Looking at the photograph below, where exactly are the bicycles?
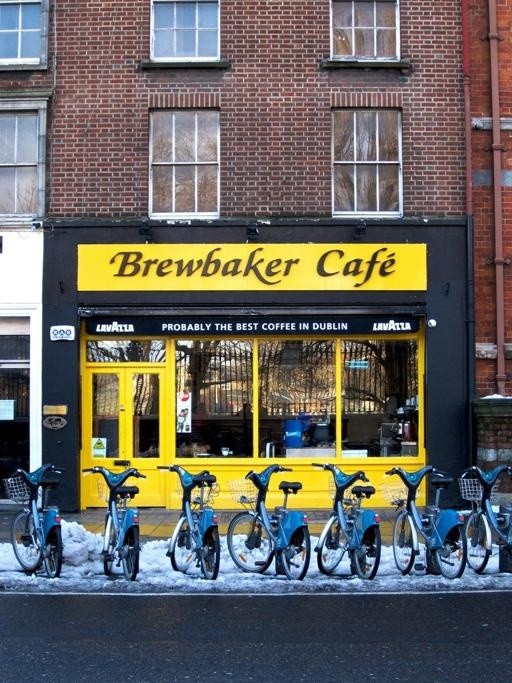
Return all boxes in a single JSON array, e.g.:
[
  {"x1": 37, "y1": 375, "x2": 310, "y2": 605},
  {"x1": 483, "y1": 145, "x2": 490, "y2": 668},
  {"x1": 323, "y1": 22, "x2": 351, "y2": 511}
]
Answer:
[
  {"x1": 387, "y1": 463, "x2": 468, "y2": 579},
  {"x1": 3, "y1": 462, "x2": 65, "y2": 579},
  {"x1": 158, "y1": 465, "x2": 221, "y2": 580},
  {"x1": 313, "y1": 461, "x2": 381, "y2": 580},
  {"x1": 83, "y1": 465, "x2": 146, "y2": 578},
  {"x1": 227, "y1": 464, "x2": 310, "y2": 581},
  {"x1": 459, "y1": 465, "x2": 512, "y2": 573}
]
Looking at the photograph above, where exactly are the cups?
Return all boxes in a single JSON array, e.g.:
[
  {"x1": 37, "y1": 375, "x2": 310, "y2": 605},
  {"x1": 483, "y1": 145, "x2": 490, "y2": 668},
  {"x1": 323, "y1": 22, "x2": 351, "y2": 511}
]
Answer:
[{"x1": 221, "y1": 447, "x2": 229, "y2": 457}]
